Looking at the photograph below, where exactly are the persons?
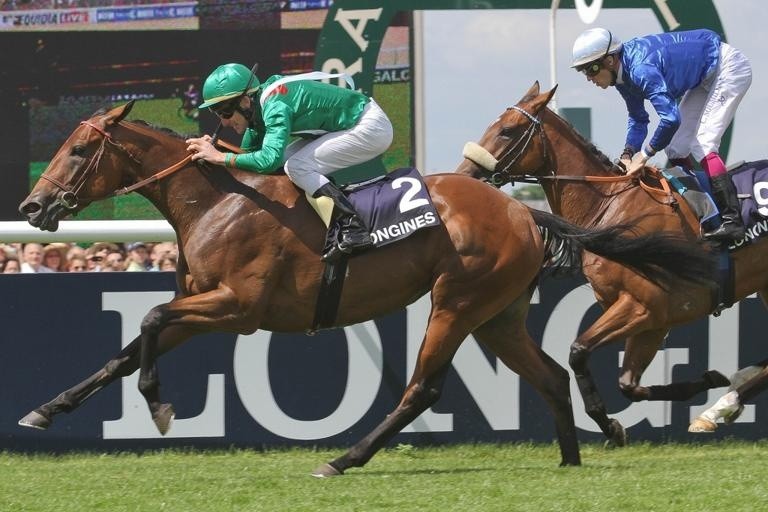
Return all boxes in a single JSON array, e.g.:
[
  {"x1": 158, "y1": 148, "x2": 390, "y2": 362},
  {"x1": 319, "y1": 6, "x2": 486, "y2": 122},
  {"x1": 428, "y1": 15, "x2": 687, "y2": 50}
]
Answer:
[
  {"x1": 183, "y1": 61, "x2": 395, "y2": 263},
  {"x1": 0, "y1": 242, "x2": 179, "y2": 274},
  {"x1": 568, "y1": 27, "x2": 752, "y2": 241}
]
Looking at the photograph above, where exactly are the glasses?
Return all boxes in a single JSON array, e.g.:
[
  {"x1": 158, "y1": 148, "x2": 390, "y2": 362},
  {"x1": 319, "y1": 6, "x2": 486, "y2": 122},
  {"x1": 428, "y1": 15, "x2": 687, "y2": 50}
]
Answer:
[
  {"x1": 213, "y1": 97, "x2": 243, "y2": 118},
  {"x1": 581, "y1": 62, "x2": 609, "y2": 78}
]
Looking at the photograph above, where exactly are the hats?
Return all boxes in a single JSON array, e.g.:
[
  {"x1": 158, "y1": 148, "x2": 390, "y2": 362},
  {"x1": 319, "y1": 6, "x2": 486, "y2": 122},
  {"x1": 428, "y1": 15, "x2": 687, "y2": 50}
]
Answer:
[{"x1": 128, "y1": 241, "x2": 146, "y2": 251}]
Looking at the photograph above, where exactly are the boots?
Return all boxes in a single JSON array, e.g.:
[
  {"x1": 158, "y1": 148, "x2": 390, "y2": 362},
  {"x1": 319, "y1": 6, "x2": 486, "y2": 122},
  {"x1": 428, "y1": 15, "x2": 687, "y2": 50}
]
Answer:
[
  {"x1": 703, "y1": 171, "x2": 746, "y2": 241},
  {"x1": 312, "y1": 182, "x2": 374, "y2": 264}
]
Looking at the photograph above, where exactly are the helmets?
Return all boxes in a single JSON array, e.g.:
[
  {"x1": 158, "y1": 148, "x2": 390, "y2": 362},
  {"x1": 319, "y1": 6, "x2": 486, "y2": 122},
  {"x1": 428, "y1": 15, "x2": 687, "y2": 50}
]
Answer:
[
  {"x1": 199, "y1": 63, "x2": 260, "y2": 109},
  {"x1": 570, "y1": 28, "x2": 622, "y2": 68}
]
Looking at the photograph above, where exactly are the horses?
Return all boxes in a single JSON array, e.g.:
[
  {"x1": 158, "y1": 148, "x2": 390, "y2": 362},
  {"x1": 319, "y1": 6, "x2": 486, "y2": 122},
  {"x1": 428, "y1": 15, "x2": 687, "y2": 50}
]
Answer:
[
  {"x1": 18, "y1": 98, "x2": 739, "y2": 479},
  {"x1": 452, "y1": 80, "x2": 768, "y2": 449}
]
